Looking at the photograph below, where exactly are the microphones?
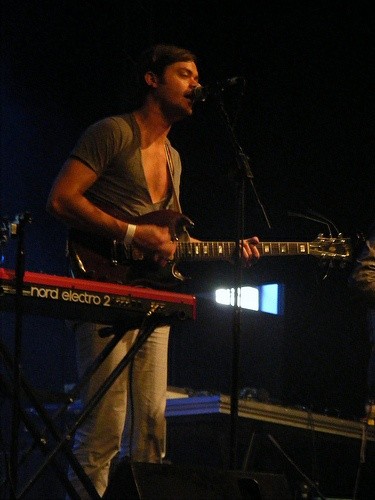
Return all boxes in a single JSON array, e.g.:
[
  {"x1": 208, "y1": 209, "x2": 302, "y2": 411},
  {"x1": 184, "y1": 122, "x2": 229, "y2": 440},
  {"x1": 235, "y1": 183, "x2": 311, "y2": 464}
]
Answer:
[
  {"x1": 8, "y1": 210, "x2": 32, "y2": 228},
  {"x1": 192, "y1": 77, "x2": 239, "y2": 101}
]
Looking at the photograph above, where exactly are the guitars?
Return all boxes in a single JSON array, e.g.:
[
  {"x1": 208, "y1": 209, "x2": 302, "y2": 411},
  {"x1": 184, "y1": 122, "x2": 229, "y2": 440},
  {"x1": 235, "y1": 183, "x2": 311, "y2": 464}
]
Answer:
[{"x1": 68, "y1": 206, "x2": 355, "y2": 282}]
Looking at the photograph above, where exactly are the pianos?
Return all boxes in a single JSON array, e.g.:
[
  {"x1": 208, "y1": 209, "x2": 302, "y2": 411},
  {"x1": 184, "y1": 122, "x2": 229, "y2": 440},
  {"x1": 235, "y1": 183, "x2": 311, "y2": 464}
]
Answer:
[{"x1": 0, "y1": 266, "x2": 209, "y2": 325}]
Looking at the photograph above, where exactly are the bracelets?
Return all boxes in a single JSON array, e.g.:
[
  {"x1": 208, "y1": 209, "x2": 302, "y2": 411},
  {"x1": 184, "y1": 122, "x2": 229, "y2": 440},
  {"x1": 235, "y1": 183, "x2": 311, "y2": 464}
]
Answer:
[{"x1": 124, "y1": 224, "x2": 136, "y2": 244}]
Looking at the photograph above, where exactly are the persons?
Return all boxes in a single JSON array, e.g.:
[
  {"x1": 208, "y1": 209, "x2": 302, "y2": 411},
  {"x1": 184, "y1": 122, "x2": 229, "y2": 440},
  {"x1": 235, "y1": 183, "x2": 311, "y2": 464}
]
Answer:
[{"x1": 46, "y1": 45, "x2": 261, "y2": 500}]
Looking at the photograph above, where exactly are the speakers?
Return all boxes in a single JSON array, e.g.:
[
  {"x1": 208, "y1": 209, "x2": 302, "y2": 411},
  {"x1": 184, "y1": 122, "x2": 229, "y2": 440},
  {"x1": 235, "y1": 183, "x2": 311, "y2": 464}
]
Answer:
[{"x1": 101, "y1": 460, "x2": 292, "y2": 500}]
